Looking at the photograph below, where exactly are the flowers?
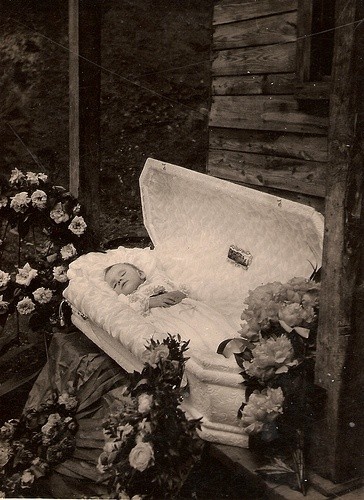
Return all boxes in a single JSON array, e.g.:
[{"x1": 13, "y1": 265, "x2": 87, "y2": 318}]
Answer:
[
  {"x1": 229, "y1": 277, "x2": 320, "y2": 446},
  {"x1": 93, "y1": 332, "x2": 204, "y2": 498},
  {"x1": 0, "y1": 168, "x2": 90, "y2": 342},
  {"x1": 0, "y1": 389, "x2": 81, "y2": 500}
]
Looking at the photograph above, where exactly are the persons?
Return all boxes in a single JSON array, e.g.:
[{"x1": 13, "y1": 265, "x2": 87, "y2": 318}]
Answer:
[{"x1": 102, "y1": 262, "x2": 247, "y2": 360}]
